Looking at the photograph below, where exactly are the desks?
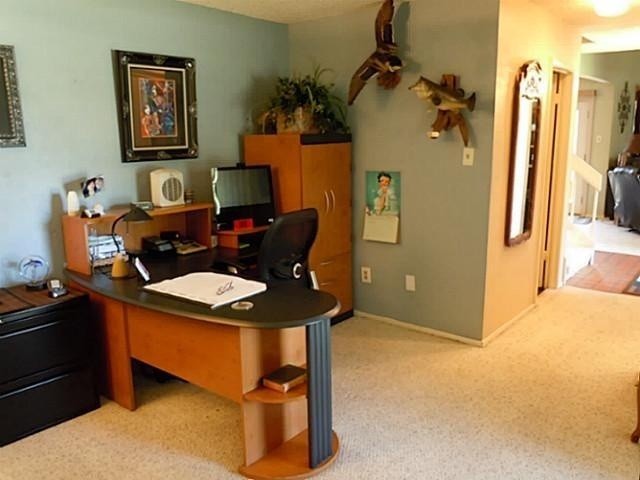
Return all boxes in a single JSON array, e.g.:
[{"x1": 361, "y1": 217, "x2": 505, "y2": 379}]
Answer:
[{"x1": 53, "y1": 191, "x2": 342, "y2": 479}]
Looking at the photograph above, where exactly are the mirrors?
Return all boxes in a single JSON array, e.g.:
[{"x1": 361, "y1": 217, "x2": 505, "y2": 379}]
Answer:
[{"x1": 504, "y1": 58, "x2": 547, "y2": 246}]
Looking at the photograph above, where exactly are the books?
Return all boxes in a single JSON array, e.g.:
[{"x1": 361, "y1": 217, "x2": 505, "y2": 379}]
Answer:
[
  {"x1": 141, "y1": 271, "x2": 267, "y2": 311},
  {"x1": 261, "y1": 364, "x2": 306, "y2": 393}
]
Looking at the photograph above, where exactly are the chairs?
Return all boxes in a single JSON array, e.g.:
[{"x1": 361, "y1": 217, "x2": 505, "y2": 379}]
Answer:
[{"x1": 227, "y1": 207, "x2": 321, "y2": 293}]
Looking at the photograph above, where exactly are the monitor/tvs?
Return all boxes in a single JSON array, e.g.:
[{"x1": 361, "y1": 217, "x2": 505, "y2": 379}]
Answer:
[{"x1": 211, "y1": 164, "x2": 275, "y2": 225}]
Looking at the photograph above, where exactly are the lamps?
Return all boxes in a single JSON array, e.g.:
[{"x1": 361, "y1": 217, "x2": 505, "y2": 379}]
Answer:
[{"x1": 109, "y1": 202, "x2": 157, "y2": 278}]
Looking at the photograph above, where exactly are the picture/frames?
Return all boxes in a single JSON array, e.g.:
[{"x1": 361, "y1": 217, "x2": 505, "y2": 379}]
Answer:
[
  {"x1": 0, "y1": 41, "x2": 29, "y2": 152},
  {"x1": 112, "y1": 46, "x2": 202, "y2": 165}
]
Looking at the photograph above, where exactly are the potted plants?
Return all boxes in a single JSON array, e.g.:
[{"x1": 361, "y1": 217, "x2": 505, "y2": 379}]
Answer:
[{"x1": 265, "y1": 61, "x2": 350, "y2": 138}]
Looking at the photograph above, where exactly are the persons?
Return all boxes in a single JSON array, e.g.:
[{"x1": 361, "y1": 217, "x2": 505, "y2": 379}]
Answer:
[{"x1": 372, "y1": 171, "x2": 395, "y2": 216}]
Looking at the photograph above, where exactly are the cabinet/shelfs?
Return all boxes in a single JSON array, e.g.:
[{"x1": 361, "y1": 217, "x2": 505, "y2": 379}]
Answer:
[
  {"x1": 1, "y1": 280, "x2": 103, "y2": 449},
  {"x1": 212, "y1": 223, "x2": 272, "y2": 260},
  {"x1": 307, "y1": 256, "x2": 356, "y2": 327},
  {"x1": 239, "y1": 130, "x2": 355, "y2": 261}
]
